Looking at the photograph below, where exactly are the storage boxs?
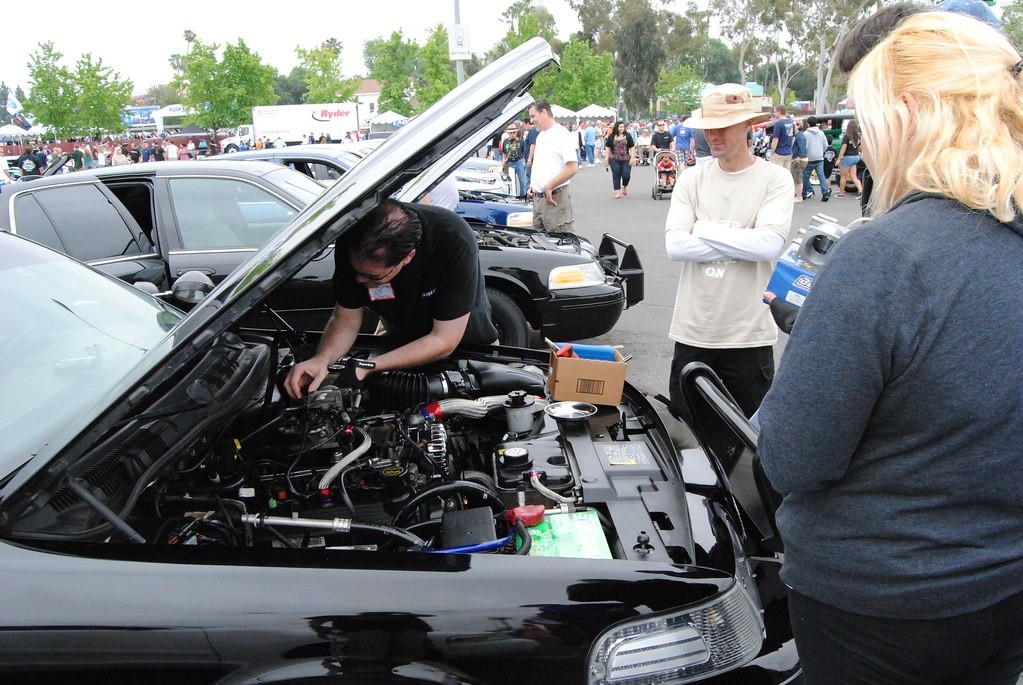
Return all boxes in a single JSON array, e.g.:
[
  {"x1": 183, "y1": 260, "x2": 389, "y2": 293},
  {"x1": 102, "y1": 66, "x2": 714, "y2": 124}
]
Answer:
[
  {"x1": 548, "y1": 342, "x2": 627, "y2": 406},
  {"x1": 761, "y1": 237, "x2": 817, "y2": 310}
]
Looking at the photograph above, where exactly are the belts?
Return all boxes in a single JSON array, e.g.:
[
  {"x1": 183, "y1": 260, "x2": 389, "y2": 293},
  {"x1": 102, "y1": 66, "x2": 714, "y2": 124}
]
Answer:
[{"x1": 534, "y1": 185, "x2": 567, "y2": 198}]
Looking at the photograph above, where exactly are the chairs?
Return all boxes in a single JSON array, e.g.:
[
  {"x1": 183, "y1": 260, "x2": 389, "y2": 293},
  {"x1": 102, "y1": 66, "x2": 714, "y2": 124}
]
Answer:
[{"x1": 182, "y1": 205, "x2": 243, "y2": 249}]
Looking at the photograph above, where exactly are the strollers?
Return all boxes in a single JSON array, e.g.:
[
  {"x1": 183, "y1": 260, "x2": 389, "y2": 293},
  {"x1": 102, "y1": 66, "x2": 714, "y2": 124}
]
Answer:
[{"x1": 651, "y1": 150, "x2": 679, "y2": 201}]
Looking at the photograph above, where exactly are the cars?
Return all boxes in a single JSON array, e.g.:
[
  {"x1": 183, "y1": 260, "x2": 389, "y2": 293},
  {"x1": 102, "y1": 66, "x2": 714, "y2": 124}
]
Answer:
[{"x1": 0, "y1": 34, "x2": 804, "y2": 685}]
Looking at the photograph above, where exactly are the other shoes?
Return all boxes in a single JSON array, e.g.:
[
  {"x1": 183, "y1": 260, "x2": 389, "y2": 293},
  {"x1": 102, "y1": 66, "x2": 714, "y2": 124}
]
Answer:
[
  {"x1": 835, "y1": 194, "x2": 844, "y2": 199},
  {"x1": 622, "y1": 188, "x2": 627, "y2": 195},
  {"x1": 577, "y1": 158, "x2": 603, "y2": 170},
  {"x1": 614, "y1": 194, "x2": 620, "y2": 199},
  {"x1": 854, "y1": 191, "x2": 862, "y2": 200},
  {"x1": 793, "y1": 187, "x2": 834, "y2": 204}
]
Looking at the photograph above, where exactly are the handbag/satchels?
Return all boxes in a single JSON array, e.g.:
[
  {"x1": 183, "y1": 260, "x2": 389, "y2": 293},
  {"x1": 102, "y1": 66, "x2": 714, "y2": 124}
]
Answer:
[{"x1": 501, "y1": 160, "x2": 508, "y2": 174}]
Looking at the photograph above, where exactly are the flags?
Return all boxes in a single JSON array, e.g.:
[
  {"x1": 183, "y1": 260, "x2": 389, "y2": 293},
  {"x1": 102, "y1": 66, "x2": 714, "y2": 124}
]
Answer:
[{"x1": 6, "y1": 93, "x2": 32, "y2": 131}]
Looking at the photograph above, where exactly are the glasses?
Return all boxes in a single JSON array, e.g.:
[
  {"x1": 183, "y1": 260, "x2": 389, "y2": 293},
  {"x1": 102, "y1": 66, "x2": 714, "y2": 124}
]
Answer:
[
  {"x1": 658, "y1": 124, "x2": 666, "y2": 126},
  {"x1": 345, "y1": 267, "x2": 396, "y2": 281}
]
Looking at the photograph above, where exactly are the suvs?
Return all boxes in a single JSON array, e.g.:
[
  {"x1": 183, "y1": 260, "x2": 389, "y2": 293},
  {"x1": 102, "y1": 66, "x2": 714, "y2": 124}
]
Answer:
[{"x1": 804, "y1": 114, "x2": 863, "y2": 193}]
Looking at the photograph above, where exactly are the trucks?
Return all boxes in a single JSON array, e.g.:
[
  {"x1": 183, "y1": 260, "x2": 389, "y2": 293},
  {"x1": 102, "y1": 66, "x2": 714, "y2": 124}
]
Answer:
[{"x1": 219, "y1": 101, "x2": 358, "y2": 153}]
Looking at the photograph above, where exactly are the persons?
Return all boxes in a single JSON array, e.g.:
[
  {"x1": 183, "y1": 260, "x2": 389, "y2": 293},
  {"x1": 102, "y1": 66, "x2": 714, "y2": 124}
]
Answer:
[
  {"x1": 527, "y1": 100, "x2": 579, "y2": 234},
  {"x1": 303, "y1": 129, "x2": 370, "y2": 145},
  {"x1": 417, "y1": 171, "x2": 460, "y2": 210},
  {"x1": 756, "y1": 10, "x2": 1023, "y2": 685},
  {"x1": 284, "y1": 198, "x2": 499, "y2": 402},
  {"x1": 770, "y1": 104, "x2": 795, "y2": 171},
  {"x1": 523, "y1": 565, "x2": 733, "y2": 654},
  {"x1": 665, "y1": 83, "x2": 794, "y2": 472},
  {"x1": 486, "y1": 115, "x2": 865, "y2": 203},
  {"x1": 0, "y1": 126, "x2": 286, "y2": 186},
  {"x1": 838, "y1": 0, "x2": 1002, "y2": 83}
]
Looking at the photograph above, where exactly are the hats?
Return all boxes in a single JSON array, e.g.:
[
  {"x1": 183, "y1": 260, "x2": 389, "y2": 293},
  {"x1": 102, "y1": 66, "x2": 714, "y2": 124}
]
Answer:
[
  {"x1": 681, "y1": 83, "x2": 772, "y2": 130},
  {"x1": 506, "y1": 123, "x2": 520, "y2": 132}
]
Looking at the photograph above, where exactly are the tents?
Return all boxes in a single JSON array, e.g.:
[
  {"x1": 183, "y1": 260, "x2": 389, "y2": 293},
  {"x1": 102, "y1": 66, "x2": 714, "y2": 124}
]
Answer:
[
  {"x1": 0, "y1": 112, "x2": 106, "y2": 144},
  {"x1": 549, "y1": 104, "x2": 617, "y2": 126},
  {"x1": 171, "y1": 123, "x2": 214, "y2": 137},
  {"x1": 369, "y1": 111, "x2": 408, "y2": 133}
]
